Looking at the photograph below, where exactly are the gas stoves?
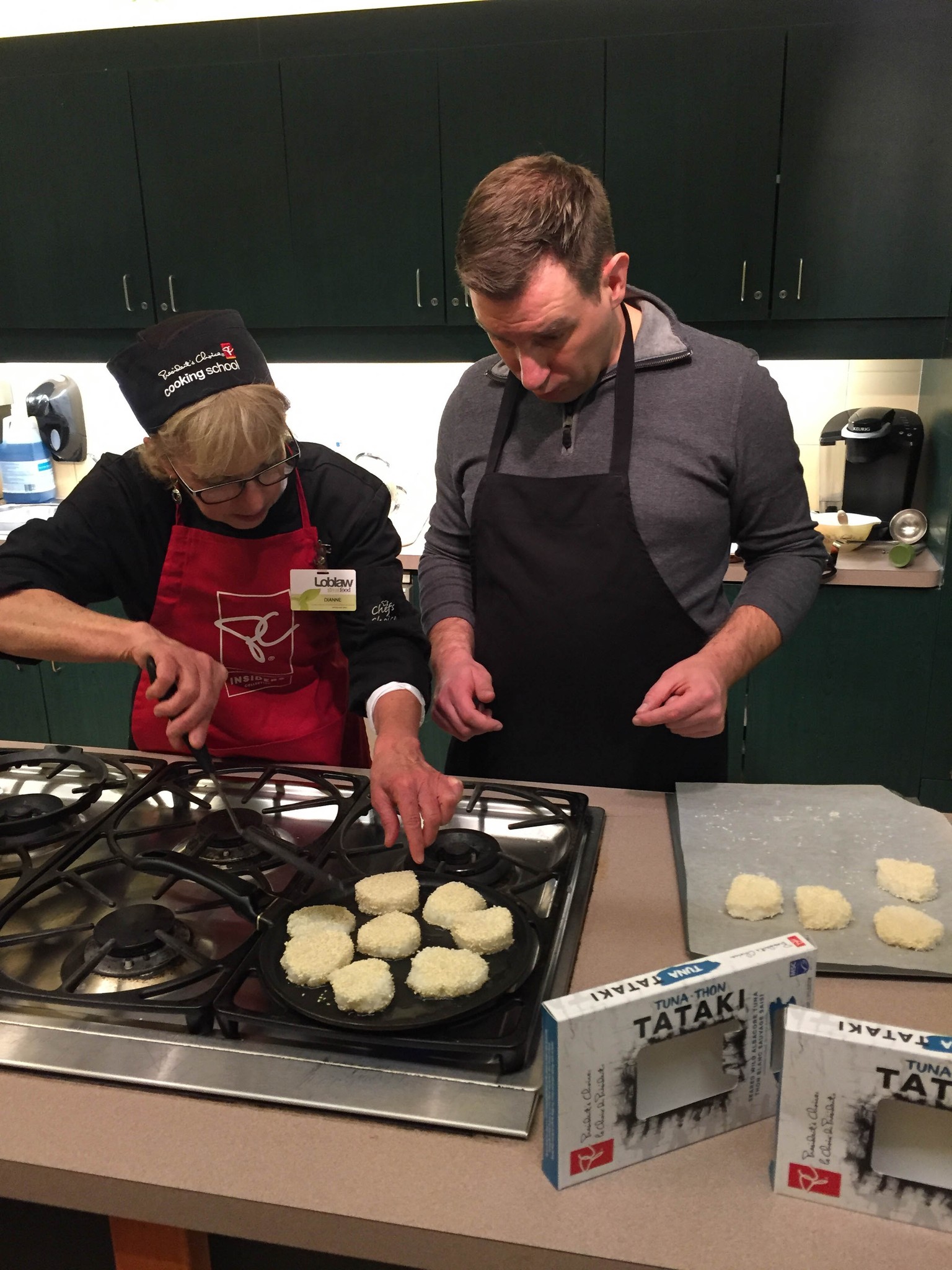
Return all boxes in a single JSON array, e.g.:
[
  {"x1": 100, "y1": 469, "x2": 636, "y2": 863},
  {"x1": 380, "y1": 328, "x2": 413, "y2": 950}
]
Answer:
[{"x1": 0, "y1": 731, "x2": 618, "y2": 1152}]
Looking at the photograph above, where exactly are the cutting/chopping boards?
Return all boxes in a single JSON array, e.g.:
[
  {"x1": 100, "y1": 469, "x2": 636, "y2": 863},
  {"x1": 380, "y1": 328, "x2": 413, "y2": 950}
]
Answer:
[{"x1": 667, "y1": 786, "x2": 952, "y2": 984}]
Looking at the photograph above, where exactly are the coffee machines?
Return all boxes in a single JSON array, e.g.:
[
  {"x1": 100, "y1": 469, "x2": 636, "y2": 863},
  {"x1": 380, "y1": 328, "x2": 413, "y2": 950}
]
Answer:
[{"x1": 819, "y1": 405, "x2": 925, "y2": 540}]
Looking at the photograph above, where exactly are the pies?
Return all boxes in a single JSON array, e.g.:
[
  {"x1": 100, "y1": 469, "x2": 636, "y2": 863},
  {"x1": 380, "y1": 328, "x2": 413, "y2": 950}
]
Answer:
[
  {"x1": 280, "y1": 867, "x2": 514, "y2": 1015},
  {"x1": 728, "y1": 854, "x2": 947, "y2": 953}
]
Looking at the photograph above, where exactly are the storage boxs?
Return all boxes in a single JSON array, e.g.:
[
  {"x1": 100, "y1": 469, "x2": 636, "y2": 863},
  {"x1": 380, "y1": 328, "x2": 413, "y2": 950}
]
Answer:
[
  {"x1": 773, "y1": 1001, "x2": 952, "y2": 1235},
  {"x1": 540, "y1": 932, "x2": 817, "y2": 1193}
]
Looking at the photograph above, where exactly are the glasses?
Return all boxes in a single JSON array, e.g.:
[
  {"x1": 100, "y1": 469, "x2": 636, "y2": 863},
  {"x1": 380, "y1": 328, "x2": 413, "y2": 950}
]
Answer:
[{"x1": 163, "y1": 422, "x2": 303, "y2": 504}]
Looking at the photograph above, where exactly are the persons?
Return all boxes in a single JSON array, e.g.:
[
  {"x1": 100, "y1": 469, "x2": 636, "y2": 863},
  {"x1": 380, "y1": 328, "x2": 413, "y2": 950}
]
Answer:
[
  {"x1": 0, "y1": 307, "x2": 466, "y2": 864},
  {"x1": 415, "y1": 152, "x2": 829, "y2": 791}
]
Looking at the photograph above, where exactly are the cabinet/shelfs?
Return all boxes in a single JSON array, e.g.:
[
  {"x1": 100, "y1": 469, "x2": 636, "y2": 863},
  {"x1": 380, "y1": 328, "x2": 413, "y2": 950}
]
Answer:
[
  {"x1": 1, "y1": 595, "x2": 139, "y2": 749},
  {"x1": 603, "y1": 16, "x2": 951, "y2": 322},
  {"x1": 0, "y1": 58, "x2": 298, "y2": 331},
  {"x1": 277, "y1": 36, "x2": 604, "y2": 329},
  {"x1": 717, "y1": 580, "x2": 942, "y2": 809}
]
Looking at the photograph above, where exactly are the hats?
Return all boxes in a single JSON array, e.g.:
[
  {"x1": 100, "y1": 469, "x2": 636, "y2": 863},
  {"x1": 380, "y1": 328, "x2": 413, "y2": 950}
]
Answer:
[{"x1": 104, "y1": 310, "x2": 275, "y2": 436}]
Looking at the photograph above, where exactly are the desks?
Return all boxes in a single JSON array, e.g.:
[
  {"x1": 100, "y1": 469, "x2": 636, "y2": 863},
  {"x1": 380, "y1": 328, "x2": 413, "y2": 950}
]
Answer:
[{"x1": 1, "y1": 740, "x2": 951, "y2": 1270}]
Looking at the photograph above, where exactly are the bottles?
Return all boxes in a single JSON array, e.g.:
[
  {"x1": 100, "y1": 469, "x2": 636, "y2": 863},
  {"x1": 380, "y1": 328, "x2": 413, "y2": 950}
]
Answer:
[{"x1": 0, "y1": 403, "x2": 58, "y2": 505}]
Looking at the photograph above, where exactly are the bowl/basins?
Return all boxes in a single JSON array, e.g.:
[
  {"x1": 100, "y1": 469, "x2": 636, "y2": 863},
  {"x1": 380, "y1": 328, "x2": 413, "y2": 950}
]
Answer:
[{"x1": 810, "y1": 508, "x2": 884, "y2": 554}]
